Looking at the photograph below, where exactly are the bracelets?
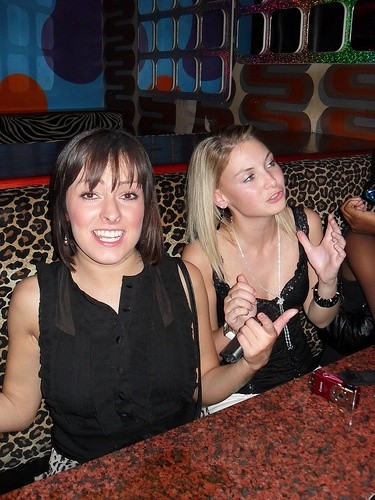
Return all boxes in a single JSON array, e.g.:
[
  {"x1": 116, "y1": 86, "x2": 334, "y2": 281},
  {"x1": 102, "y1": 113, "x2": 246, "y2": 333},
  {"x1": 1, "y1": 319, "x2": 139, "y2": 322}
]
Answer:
[{"x1": 313, "y1": 282, "x2": 340, "y2": 307}]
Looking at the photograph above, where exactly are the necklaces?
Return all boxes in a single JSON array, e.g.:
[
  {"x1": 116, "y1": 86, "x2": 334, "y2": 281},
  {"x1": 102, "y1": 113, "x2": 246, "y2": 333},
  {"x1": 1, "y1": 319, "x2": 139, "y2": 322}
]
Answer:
[{"x1": 230, "y1": 216, "x2": 294, "y2": 350}]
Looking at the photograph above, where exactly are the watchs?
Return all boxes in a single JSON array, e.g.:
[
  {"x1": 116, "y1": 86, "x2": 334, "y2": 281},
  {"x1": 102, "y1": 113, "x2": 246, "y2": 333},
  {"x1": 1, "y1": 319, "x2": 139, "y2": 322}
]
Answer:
[{"x1": 224, "y1": 322, "x2": 236, "y2": 340}]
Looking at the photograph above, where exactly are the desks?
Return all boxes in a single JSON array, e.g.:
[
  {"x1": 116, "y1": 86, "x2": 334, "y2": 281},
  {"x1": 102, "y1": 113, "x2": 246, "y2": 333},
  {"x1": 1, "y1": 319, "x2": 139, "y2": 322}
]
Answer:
[{"x1": 0, "y1": 343, "x2": 375, "y2": 500}]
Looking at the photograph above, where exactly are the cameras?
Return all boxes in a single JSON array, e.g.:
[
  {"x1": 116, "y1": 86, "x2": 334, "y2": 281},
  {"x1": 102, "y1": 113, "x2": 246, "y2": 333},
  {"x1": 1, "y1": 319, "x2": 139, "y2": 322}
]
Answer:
[{"x1": 308, "y1": 369, "x2": 361, "y2": 411}]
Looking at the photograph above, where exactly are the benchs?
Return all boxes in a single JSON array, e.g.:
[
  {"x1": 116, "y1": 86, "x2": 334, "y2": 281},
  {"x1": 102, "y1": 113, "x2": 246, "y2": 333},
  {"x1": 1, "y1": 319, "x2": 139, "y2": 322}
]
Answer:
[{"x1": 0, "y1": 154, "x2": 375, "y2": 493}]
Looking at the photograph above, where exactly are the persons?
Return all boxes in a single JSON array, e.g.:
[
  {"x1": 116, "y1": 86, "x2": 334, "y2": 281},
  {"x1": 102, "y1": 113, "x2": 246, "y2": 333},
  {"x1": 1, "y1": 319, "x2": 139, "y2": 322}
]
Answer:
[
  {"x1": 181, "y1": 125, "x2": 347, "y2": 415},
  {"x1": 0, "y1": 128, "x2": 299, "y2": 477},
  {"x1": 342, "y1": 197, "x2": 375, "y2": 316}
]
